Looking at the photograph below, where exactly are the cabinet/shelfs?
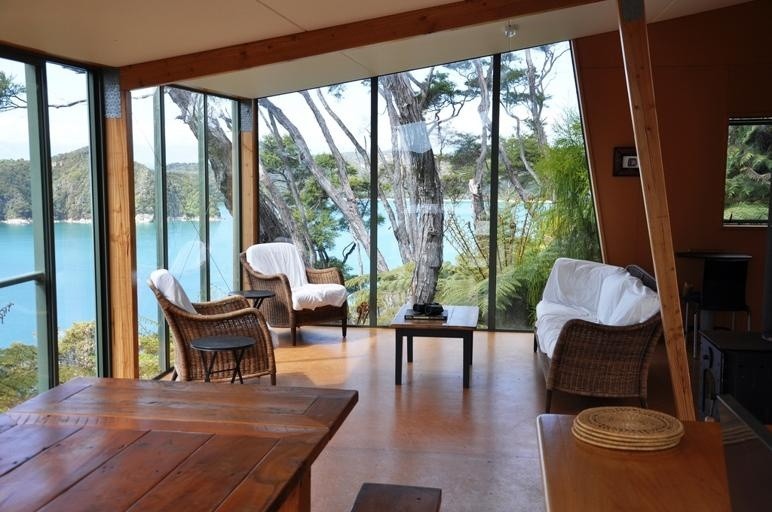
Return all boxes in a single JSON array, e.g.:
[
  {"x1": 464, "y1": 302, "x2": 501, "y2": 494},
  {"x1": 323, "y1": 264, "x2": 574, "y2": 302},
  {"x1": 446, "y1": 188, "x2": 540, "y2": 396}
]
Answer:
[{"x1": 697, "y1": 329, "x2": 772, "y2": 423}]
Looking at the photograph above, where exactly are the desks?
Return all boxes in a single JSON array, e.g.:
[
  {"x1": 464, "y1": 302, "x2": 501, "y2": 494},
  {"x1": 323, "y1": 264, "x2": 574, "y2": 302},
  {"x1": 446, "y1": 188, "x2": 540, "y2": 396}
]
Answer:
[
  {"x1": 537, "y1": 414, "x2": 732, "y2": 512},
  {"x1": 2, "y1": 371, "x2": 357, "y2": 512}
]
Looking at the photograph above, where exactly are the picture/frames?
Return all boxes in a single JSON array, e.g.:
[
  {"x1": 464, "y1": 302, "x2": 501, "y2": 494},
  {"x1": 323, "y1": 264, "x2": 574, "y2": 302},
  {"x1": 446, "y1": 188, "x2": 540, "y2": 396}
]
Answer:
[{"x1": 613, "y1": 147, "x2": 640, "y2": 178}]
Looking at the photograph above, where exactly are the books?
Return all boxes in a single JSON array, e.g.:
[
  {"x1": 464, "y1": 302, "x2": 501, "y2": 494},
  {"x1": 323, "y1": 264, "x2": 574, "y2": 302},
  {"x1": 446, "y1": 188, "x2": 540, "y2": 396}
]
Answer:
[{"x1": 404, "y1": 309, "x2": 448, "y2": 320}]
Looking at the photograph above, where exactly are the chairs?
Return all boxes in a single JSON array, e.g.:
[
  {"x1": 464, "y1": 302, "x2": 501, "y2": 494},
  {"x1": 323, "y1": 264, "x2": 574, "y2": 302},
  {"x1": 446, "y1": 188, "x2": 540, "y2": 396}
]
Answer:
[
  {"x1": 147, "y1": 242, "x2": 348, "y2": 387},
  {"x1": 675, "y1": 251, "x2": 753, "y2": 360}
]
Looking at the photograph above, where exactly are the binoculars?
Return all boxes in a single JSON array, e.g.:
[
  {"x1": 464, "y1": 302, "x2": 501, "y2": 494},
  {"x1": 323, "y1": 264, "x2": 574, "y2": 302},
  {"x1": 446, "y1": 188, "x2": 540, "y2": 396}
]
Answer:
[{"x1": 413, "y1": 303, "x2": 443, "y2": 316}]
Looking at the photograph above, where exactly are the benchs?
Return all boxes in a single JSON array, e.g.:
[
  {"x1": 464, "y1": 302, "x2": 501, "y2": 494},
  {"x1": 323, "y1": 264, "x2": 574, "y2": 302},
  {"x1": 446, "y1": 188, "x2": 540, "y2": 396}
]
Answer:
[{"x1": 533, "y1": 266, "x2": 664, "y2": 414}]
